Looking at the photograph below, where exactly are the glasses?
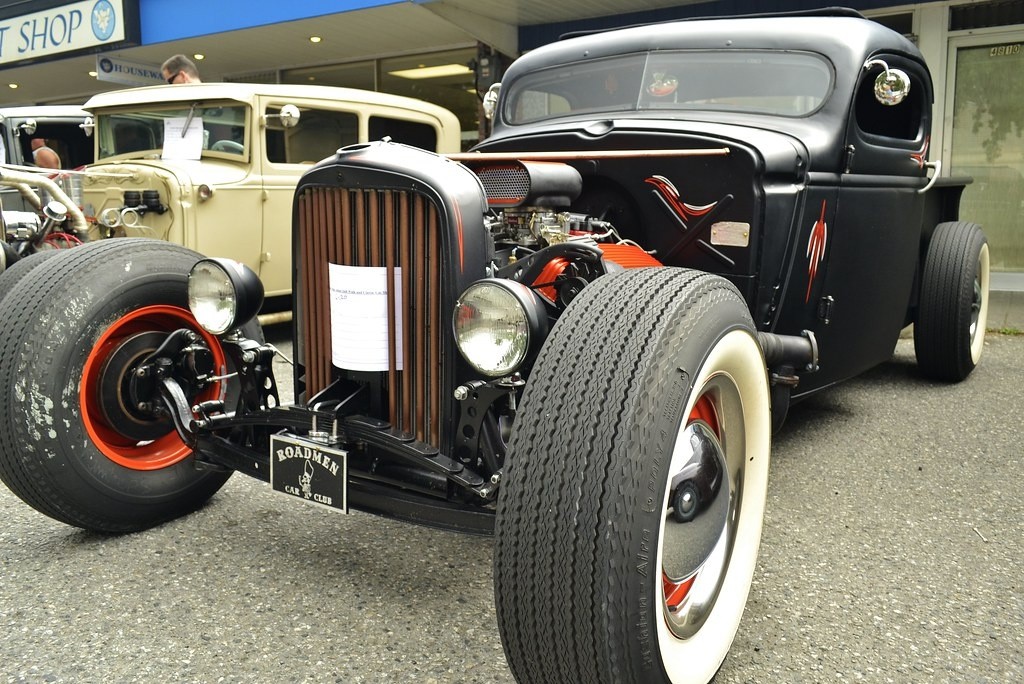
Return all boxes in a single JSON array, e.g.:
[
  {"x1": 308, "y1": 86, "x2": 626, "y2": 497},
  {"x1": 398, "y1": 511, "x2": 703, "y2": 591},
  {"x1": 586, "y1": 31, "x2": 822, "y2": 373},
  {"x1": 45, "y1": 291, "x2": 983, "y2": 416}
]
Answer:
[{"x1": 166, "y1": 72, "x2": 180, "y2": 84}]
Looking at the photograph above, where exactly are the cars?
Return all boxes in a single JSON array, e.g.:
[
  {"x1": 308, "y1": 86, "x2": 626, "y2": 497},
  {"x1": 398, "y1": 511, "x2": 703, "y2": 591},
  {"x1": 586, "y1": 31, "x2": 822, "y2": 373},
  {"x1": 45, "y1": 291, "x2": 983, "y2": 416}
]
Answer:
[
  {"x1": 0, "y1": 80, "x2": 463, "y2": 352},
  {"x1": 0, "y1": 3, "x2": 994, "y2": 683},
  {"x1": 0, "y1": 104, "x2": 167, "y2": 218}
]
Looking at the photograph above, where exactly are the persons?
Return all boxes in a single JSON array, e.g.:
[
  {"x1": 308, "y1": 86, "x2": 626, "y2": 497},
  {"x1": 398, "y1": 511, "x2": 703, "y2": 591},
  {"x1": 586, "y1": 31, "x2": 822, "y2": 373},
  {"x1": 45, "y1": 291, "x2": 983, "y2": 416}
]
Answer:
[
  {"x1": 161, "y1": 54, "x2": 201, "y2": 85},
  {"x1": 30, "y1": 139, "x2": 61, "y2": 170}
]
